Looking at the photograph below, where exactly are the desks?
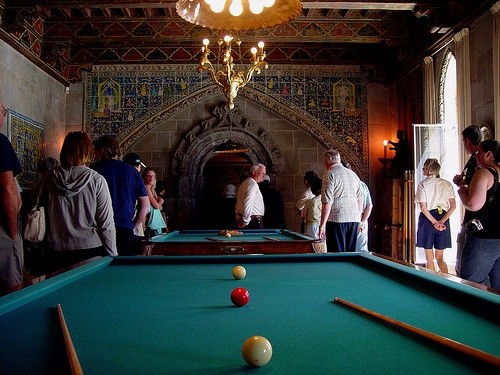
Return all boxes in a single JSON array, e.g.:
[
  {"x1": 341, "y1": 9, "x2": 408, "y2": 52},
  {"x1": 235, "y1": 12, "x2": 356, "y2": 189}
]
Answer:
[
  {"x1": 146, "y1": 231, "x2": 318, "y2": 255},
  {"x1": 0, "y1": 254, "x2": 500, "y2": 375}
]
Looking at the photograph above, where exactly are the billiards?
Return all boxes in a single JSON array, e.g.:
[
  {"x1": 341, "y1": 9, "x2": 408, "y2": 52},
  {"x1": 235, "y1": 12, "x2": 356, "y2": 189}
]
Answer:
[
  {"x1": 243, "y1": 336, "x2": 272, "y2": 366},
  {"x1": 233, "y1": 266, "x2": 246, "y2": 279},
  {"x1": 226, "y1": 233, "x2": 231, "y2": 238},
  {"x1": 231, "y1": 287, "x2": 250, "y2": 306},
  {"x1": 221, "y1": 230, "x2": 240, "y2": 233}
]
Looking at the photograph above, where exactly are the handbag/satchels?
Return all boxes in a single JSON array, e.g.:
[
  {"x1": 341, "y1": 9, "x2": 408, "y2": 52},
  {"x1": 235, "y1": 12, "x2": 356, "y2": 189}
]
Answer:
[{"x1": 24, "y1": 205, "x2": 45, "y2": 242}]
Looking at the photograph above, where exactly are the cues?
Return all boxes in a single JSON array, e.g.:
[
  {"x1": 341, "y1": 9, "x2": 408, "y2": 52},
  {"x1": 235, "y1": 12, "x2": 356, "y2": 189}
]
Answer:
[
  {"x1": 57, "y1": 303, "x2": 84, "y2": 375},
  {"x1": 206, "y1": 237, "x2": 224, "y2": 241},
  {"x1": 263, "y1": 236, "x2": 281, "y2": 241},
  {"x1": 402, "y1": 170, "x2": 414, "y2": 263},
  {"x1": 334, "y1": 296, "x2": 500, "y2": 373}
]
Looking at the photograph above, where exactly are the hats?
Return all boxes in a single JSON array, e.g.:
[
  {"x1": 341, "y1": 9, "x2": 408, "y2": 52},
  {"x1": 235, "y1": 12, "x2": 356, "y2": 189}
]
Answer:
[{"x1": 123, "y1": 153, "x2": 146, "y2": 168}]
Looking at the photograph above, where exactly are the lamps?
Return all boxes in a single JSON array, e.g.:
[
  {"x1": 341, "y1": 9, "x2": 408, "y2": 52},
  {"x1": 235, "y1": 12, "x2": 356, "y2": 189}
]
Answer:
[
  {"x1": 176, "y1": 0, "x2": 303, "y2": 31},
  {"x1": 196, "y1": 30, "x2": 268, "y2": 110}
]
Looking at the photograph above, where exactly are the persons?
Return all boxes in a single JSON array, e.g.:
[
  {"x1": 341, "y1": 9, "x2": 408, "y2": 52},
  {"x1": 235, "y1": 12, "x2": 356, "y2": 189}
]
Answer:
[
  {"x1": 457, "y1": 140, "x2": 500, "y2": 289},
  {"x1": 123, "y1": 153, "x2": 146, "y2": 242},
  {"x1": 142, "y1": 167, "x2": 172, "y2": 255},
  {"x1": 235, "y1": 163, "x2": 266, "y2": 229},
  {"x1": 0, "y1": 105, "x2": 24, "y2": 285},
  {"x1": 304, "y1": 178, "x2": 323, "y2": 243},
  {"x1": 42, "y1": 132, "x2": 119, "y2": 268},
  {"x1": 295, "y1": 170, "x2": 318, "y2": 233},
  {"x1": 414, "y1": 159, "x2": 457, "y2": 273},
  {"x1": 453, "y1": 125, "x2": 480, "y2": 277},
  {"x1": 221, "y1": 184, "x2": 237, "y2": 229},
  {"x1": 341, "y1": 162, "x2": 373, "y2": 252},
  {"x1": 260, "y1": 175, "x2": 287, "y2": 229},
  {"x1": 89, "y1": 135, "x2": 150, "y2": 256},
  {"x1": 318, "y1": 150, "x2": 365, "y2": 253}
]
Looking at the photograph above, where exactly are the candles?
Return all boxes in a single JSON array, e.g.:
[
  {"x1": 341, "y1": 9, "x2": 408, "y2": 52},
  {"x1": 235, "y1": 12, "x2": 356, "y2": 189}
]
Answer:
[{"x1": 383, "y1": 140, "x2": 388, "y2": 158}]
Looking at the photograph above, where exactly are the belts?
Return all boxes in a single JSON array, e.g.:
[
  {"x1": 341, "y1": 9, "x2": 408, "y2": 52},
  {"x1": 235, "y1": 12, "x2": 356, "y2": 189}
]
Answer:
[{"x1": 250, "y1": 215, "x2": 263, "y2": 219}]
